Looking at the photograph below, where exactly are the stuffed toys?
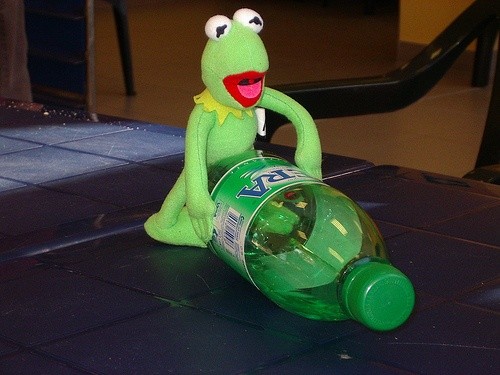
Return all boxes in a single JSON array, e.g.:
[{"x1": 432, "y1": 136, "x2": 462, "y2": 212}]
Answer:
[{"x1": 144, "y1": 9, "x2": 323, "y2": 247}]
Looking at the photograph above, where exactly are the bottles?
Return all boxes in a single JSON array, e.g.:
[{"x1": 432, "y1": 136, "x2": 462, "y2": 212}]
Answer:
[{"x1": 202, "y1": 148, "x2": 416, "y2": 332}]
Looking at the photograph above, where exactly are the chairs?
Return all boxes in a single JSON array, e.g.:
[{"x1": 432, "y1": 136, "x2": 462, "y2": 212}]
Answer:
[{"x1": 253, "y1": 1, "x2": 499, "y2": 185}]
